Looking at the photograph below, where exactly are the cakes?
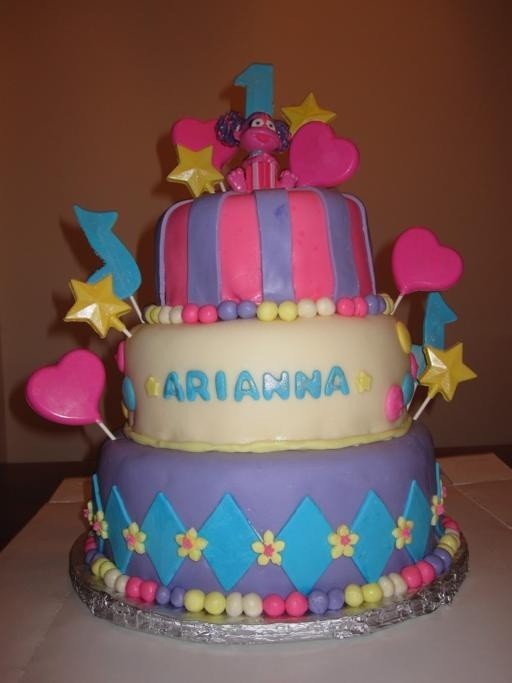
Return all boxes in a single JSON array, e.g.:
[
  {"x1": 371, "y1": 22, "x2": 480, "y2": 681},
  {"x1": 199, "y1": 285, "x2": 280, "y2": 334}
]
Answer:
[{"x1": 24, "y1": 60, "x2": 479, "y2": 620}]
